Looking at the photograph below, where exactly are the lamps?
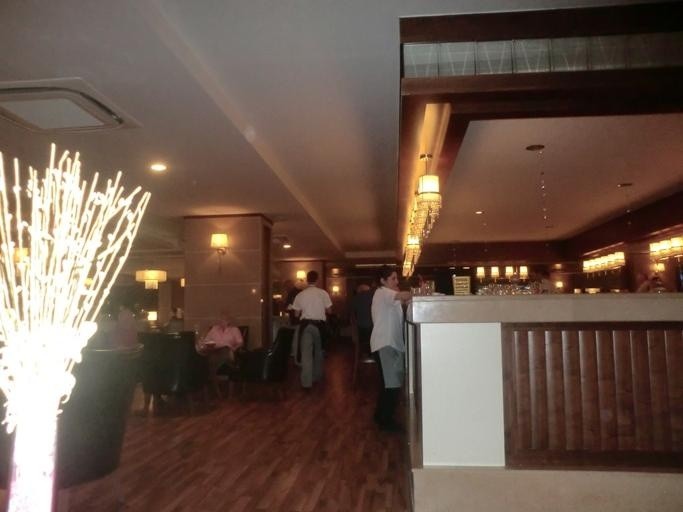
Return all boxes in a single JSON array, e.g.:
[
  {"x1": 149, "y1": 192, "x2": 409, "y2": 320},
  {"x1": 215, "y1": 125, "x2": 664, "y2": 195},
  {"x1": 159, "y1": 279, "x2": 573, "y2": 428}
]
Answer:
[
  {"x1": 582, "y1": 251, "x2": 625, "y2": 280},
  {"x1": 136, "y1": 269, "x2": 167, "y2": 290},
  {"x1": 491, "y1": 267, "x2": 500, "y2": 282},
  {"x1": 211, "y1": 233, "x2": 228, "y2": 276},
  {"x1": 649, "y1": 236, "x2": 683, "y2": 274},
  {"x1": 519, "y1": 265, "x2": 529, "y2": 282},
  {"x1": 476, "y1": 266, "x2": 485, "y2": 283},
  {"x1": 401, "y1": 153, "x2": 442, "y2": 279},
  {"x1": 505, "y1": 266, "x2": 514, "y2": 284},
  {"x1": 330, "y1": 268, "x2": 341, "y2": 278},
  {"x1": 296, "y1": 271, "x2": 306, "y2": 281}
]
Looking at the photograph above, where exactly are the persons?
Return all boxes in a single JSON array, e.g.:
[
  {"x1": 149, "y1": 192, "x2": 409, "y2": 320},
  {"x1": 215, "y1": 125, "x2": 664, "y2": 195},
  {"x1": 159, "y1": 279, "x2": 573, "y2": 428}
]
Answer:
[
  {"x1": 286, "y1": 271, "x2": 333, "y2": 390},
  {"x1": 370, "y1": 265, "x2": 414, "y2": 431},
  {"x1": 204, "y1": 311, "x2": 256, "y2": 382}
]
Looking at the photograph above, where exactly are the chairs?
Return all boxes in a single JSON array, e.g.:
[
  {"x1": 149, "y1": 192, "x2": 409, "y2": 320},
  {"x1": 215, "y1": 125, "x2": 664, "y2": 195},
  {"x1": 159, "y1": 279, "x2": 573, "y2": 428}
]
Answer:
[
  {"x1": 136, "y1": 324, "x2": 296, "y2": 405},
  {"x1": 350, "y1": 318, "x2": 378, "y2": 387}
]
